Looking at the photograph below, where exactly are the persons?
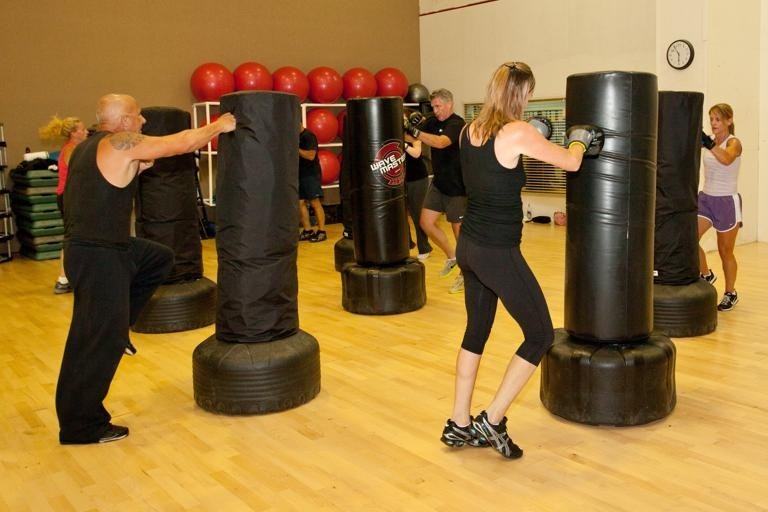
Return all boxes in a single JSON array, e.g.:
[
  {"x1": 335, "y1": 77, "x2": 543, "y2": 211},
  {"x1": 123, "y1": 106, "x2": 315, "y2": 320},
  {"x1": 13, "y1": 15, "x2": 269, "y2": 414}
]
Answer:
[
  {"x1": 55, "y1": 92, "x2": 237, "y2": 445},
  {"x1": 298, "y1": 139, "x2": 327, "y2": 242},
  {"x1": 441, "y1": 61, "x2": 600, "y2": 460},
  {"x1": 400, "y1": 108, "x2": 433, "y2": 260},
  {"x1": 404, "y1": 87, "x2": 467, "y2": 295},
  {"x1": 697, "y1": 102, "x2": 743, "y2": 312},
  {"x1": 37, "y1": 114, "x2": 89, "y2": 294}
]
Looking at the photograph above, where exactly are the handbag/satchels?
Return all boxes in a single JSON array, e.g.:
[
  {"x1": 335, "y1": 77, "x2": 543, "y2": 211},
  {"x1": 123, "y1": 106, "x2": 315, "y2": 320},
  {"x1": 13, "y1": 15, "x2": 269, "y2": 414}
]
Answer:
[
  {"x1": 524, "y1": 216, "x2": 551, "y2": 223},
  {"x1": 554, "y1": 211, "x2": 566, "y2": 226}
]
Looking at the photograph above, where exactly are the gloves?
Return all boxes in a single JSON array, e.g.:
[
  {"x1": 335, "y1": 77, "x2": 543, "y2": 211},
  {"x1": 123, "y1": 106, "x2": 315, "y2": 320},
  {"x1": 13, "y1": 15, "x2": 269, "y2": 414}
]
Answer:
[
  {"x1": 402, "y1": 114, "x2": 420, "y2": 138},
  {"x1": 564, "y1": 123, "x2": 605, "y2": 157},
  {"x1": 701, "y1": 132, "x2": 716, "y2": 150},
  {"x1": 525, "y1": 115, "x2": 553, "y2": 140},
  {"x1": 409, "y1": 111, "x2": 428, "y2": 131}
]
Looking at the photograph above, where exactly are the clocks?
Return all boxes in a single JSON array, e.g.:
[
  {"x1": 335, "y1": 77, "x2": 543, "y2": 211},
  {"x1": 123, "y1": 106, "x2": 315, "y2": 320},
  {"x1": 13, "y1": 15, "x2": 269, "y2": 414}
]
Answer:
[{"x1": 667, "y1": 38, "x2": 695, "y2": 70}]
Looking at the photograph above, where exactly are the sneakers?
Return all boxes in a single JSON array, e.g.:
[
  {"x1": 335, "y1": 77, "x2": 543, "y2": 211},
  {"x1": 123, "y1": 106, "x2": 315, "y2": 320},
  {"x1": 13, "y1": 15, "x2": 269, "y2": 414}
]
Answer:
[
  {"x1": 415, "y1": 251, "x2": 430, "y2": 260},
  {"x1": 298, "y1": 229, "x2": 315, "y2": 241},
  {"x1": 718, "y1": 288, "x2": 738, "y2": 312},
  {"x1": 448, "y1": 274, "x2": 464, "y2": 294},
  {"x1": 124, "y1": 339, "x2": 136, "y2": 357},
  {"x1": 469, "y1": 411, "x2": 522, "y2": 459},
  {"x1": 440, "y1": 415, "x2": 490, "y2": 448},
  {"x1": 54, "y1": 276, "x2": 72, "y2": 294},
  {"x1": 439, "y1": 258, "x2": 456, "y2": 277},
  {"x1": 700, "y1": 269, "x2": 716, "y2": 284},
  {"x1": 96, "y1": 424, "x2": 129, "y2": 443},
  {"x1": 309, "y1": 230, "x2": 327, "y2": 242}
]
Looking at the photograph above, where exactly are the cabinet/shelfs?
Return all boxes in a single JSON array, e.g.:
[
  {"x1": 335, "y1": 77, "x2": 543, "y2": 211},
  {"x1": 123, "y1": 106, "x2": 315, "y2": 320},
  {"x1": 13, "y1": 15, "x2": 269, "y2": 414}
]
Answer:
[{"x1": 190, "y1": 95, "x2": 437, "y2": 210}]
